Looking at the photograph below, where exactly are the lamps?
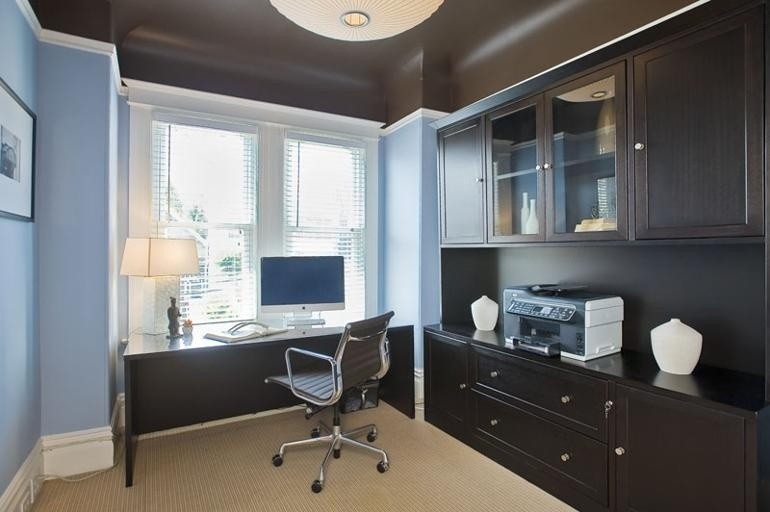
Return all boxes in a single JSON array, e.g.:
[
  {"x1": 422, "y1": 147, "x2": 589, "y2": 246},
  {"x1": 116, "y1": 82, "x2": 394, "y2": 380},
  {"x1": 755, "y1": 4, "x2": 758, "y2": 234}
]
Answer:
[
  {"x1": 269, "y1": 0, "x2": 446, "y2": 41},
  {"x1": 120, "y1": 237, "x2": 200, "y2": 336}
]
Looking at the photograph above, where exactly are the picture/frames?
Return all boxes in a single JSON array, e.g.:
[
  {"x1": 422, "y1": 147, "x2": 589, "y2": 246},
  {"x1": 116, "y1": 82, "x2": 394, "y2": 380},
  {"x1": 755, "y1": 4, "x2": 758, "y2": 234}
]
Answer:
[{"x1": 0, "y1": 77, "x2": 37, "y2": 222}]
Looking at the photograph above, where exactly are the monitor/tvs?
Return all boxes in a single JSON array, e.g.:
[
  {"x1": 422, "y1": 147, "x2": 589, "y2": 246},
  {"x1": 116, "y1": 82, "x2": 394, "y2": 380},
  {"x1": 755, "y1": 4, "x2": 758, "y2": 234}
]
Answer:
[{"x1": 260, "y1": 254, "x2": 346, "y2": 325}]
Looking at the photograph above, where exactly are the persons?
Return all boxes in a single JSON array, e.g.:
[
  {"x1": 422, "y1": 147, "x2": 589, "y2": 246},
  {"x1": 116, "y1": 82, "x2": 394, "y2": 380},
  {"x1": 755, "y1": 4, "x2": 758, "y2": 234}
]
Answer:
[{"x1": 167, "y1": 297, "x2": 181, "y2": 337}]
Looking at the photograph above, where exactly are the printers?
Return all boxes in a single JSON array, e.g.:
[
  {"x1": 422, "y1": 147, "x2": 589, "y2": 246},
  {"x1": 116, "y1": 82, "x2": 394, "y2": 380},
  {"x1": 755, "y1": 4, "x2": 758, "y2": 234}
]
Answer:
[{"x1": 502, "y1": 283, "x2": 625, "y2": 362}]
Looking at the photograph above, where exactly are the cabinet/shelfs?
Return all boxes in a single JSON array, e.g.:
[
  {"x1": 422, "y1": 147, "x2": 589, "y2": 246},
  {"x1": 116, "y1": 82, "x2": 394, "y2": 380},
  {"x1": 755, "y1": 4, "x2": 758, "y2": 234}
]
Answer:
[
  {"x1": 485, "y1": 58, "x2": 627, "y2": 246},
  {"x1": 436, "y1": 111, "x2": 485, "y2": 250},
  {"x1": 615, "y1": 380, "x2": 745, "y2": 512},
  {"x1": 469, "y1": 344, "x2": 610, "y2": 511},
  {"x1": 633, "y1": 7, "x2": 768, "y2": 245},
  {"x1": 426, "y1": 330, "x2": 466, "y2": 443}
]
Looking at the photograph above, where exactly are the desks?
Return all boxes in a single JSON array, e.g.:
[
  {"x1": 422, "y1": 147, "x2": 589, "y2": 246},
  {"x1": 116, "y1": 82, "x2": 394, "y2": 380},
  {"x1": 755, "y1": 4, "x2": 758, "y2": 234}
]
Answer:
[{"x1": 123, "y1": 313, "x2": 414, "y2": 486}]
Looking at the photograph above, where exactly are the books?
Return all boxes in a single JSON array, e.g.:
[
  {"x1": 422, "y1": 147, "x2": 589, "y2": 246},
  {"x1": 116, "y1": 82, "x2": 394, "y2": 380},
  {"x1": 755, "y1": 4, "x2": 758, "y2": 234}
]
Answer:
[
  {"x1": 204, "y1": 319, "x2": 287, "y2": 343},
  {"x1": 573, "y1": 217, "x2": 616, "y2": 232},
  {"x1": 596, "y1": 176, "x2": 616, "y2": 220}
]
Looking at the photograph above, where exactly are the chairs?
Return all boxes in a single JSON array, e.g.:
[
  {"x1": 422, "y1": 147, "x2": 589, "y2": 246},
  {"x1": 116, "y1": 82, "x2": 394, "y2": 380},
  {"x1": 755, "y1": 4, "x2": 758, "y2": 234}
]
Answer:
[{"x1": 264, "y1": 310, "x2": 394, "y2": 494}]
